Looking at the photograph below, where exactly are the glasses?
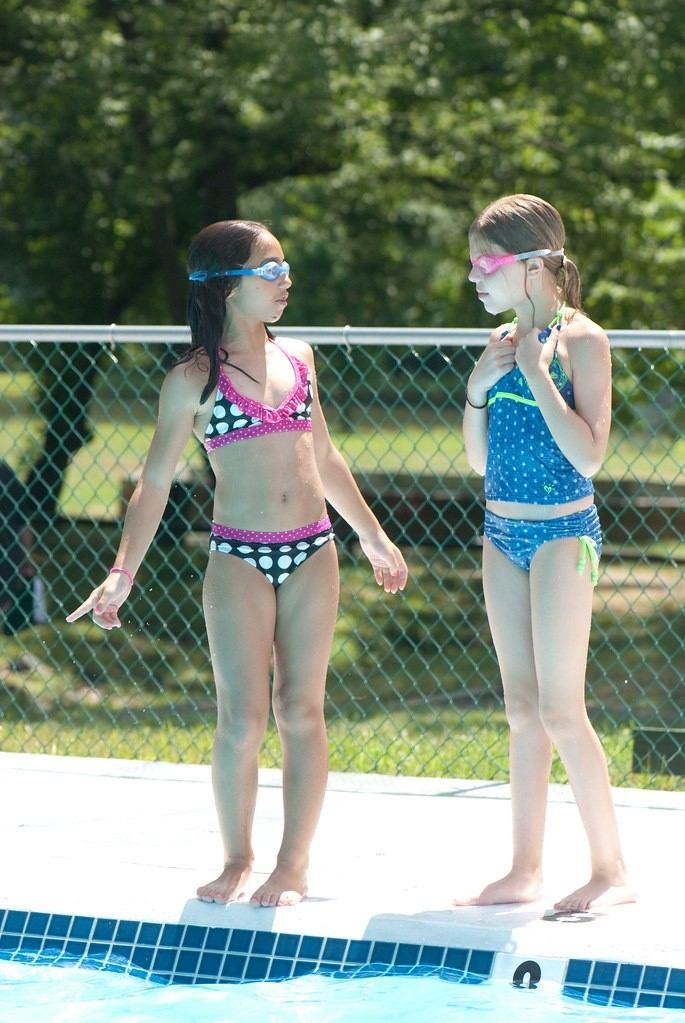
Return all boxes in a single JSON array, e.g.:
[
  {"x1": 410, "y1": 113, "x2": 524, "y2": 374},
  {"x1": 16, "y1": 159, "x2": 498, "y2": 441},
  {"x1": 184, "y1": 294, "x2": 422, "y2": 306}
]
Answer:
[
  {"x1": 188, "y1": 259, "x2": 294, "y2": 281},
  {"x1": 471, "y1": 249, "x2": 553, "y2": 276}
]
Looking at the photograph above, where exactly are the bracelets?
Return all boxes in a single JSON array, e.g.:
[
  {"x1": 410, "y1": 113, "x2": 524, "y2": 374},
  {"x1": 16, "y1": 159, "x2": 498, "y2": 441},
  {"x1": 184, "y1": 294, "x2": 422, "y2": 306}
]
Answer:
[
  {"x1": 106, "y1": 564, "x2": 137, "y2": 589},
  {"x1": 464, "y1": 383, "x2": 489, "y2": 409}
]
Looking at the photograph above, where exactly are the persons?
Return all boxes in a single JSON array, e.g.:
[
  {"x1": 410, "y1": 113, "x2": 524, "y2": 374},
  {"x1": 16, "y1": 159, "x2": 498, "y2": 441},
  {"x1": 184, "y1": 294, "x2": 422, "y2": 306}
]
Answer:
[
  {"x1": 64, "y1": 221, "x2": 411, "y2": 906},
  {"x1": 463, "y1": 193, "x2": 630, "y2": 911},
  {"x1": 0, "y1": 463, "x2": 35, "y2": 613}
]
